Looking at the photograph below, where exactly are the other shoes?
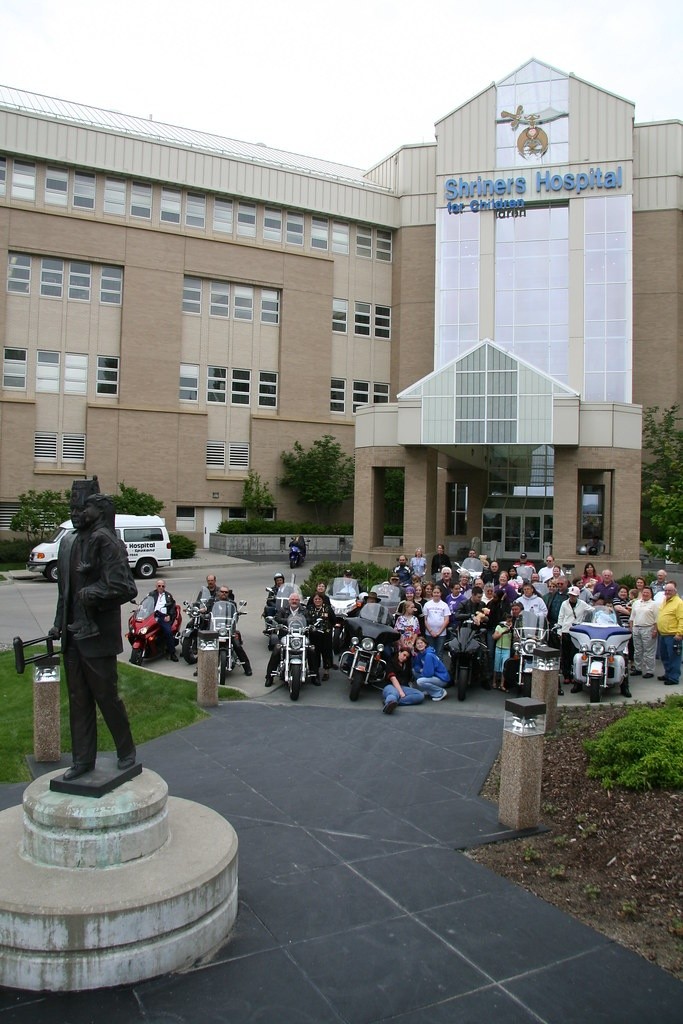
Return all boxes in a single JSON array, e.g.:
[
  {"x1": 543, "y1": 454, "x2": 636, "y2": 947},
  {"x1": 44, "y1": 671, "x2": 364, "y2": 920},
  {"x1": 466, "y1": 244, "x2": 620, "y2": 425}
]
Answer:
[
  {"x1": 432, "y1": 689, "x2": 448, "y2": 701},
  {"x1": 665, "y1": 680, "x2": 678, "y2": 685},
  {"x1": 620, "y1": 687, "x2": 631, "y2": 697},
  {"x1": 658, "y1": 676, "x2": 668, "y2": 681},
  {"x1": 170, "y1": 655, "x2": 179, "y2": 661},
  {"x1": 384, "y1": 702, "x2": 398, "y2": 714},
  {"x1": 322, "y1": 674, "x2": 329, "y2": 681},
  {"x1": 571, "y1": 682, "x2": 583, "y2": 693},
  {"x1": 265, "y1": 679, "x2": 272, "y2": 687},
  {"x1": 631, "y1": 670, "x2": 642, "y2": 676},
  {"x1": 245, "y1": 671, "x2": 252, "y2": 676},
  {"x1": 643, "y1": 673, "x2": 654, "y2": 678},
  {"x1": 564, "y1": 678, "x2": 573, "y2": 684},
  {"x1": 312, "y1": 677, "x2": 321, "y2": 686}
]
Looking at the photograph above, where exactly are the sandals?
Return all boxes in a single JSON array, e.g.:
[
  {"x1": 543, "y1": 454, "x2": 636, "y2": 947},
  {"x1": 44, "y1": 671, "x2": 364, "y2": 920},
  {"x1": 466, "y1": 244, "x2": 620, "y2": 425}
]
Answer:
[{"x1": 498, "y1": 685, "x2": 509, "y2": 692}]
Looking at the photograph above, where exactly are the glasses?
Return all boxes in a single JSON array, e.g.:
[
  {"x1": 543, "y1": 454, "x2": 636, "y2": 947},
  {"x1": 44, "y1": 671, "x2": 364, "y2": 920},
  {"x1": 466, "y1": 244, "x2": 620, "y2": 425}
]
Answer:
[
  {"x1": 158, "y1": 585, "x2": 165, "y2": 587},
  {"x1": 444, "y1": 573, "x2": 449, "y2": 574},
  {"x1": 346, "y1": 574, "x2": 351, "y2": 576},
  {"x1": 547, "y1": 560, "x2": 552, "y2": 562},
  {"x1": 221, "y1": 591, "x2": 229, "y2": 593},
  {"x1": 521, "y1": 556, "x2": 525, "y2": 558}
]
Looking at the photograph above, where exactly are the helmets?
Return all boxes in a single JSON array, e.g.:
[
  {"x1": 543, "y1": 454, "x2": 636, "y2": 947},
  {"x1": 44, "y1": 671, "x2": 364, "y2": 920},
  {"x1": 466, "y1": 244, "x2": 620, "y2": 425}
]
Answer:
[{"x1": 274, "y1": 572, "x2": 284, "y2": 579}]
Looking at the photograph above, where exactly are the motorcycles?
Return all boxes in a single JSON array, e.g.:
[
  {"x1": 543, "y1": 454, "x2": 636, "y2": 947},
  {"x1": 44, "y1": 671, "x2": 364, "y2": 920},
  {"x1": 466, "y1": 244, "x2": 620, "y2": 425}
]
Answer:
[
  {"x1": 180, "y1": 586, "x2": 216, "y2": 665},
  {"x1": 567, "y1": 623, "x2": 632, "y2": 703},
  {"x1": 453, "y1": 557, "x2": 484, "y2": 585},
  {"x1": 443, "y1": 616, "x2": 488, "y2": 700},
  {"x1": 265, "y1": 616, "x2": 330, "y2": 701},
  {"x1": 335, "y1": 603, "x2": 401, "y2": 701},
  {"x1": 499, "y1": 616, "x2": 562, "y2": 696},
  {"x1": 124, "y1": 595, "x2": 182, "y2": 665},
  {"x1": 199, "y1": 598, "x2": 248, "y2": 684}
]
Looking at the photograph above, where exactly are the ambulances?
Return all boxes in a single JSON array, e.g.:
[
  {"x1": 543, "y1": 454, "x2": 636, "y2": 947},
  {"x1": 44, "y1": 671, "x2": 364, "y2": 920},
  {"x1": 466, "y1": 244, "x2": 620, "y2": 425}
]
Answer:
[{"x1": 27, "y1": 514, "x2": 173, "y2": 583}]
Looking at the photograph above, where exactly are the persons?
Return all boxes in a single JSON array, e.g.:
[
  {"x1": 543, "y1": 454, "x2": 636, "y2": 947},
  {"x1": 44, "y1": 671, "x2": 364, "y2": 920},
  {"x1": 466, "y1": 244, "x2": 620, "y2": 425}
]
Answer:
[
  {"x1": 149, "y1": 580, "x2": 179, "y2": 662},
  {"x1": 206, "y1": 575, "x2": 219, "y2": 601},
  {"x1": 49, "y1": 476, "x2": 139, "y2": 782},
  {"x1": 629, "y1": 587, "x2": 659, "y2": 678},
  {"x1": 347, "y1": 592, "x2": 383, "y2": 617},
  {"x1": 308, "y1": 594, "x2": 337, "y2": 681},
  {"x1": 308, "y1": 581, "x2": 332, "y2": 611},
  {"x1": 389, "y1": 545, "x2": 683, "y2": 686},
  {"x1": 265, "y1": 593, "x2": 322, "y2": 687},
  {"x1": 330, "y1": 570, "x2": 362, "y2": 600},
  {"x1": 268, "y1": 573, "x2": 285, "y2": 605},
  {"x1": 193, "y1": 586, "x2": 253, "y2": 676},
  {"x1": 382, "y1": 647, "x2": 424, "y2": 716},
  {"x1": 413, "y1": 637, "x2": 450, "y2": 701},
  {"x1": 658, "y1": 583, "x2": 683, "y2": 685}
]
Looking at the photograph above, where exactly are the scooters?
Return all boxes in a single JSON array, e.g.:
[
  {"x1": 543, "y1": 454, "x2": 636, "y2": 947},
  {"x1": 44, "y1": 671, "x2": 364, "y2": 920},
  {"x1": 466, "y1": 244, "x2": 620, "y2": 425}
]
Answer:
[
  {"x1": 366, "y1": 584, "x2": 402, "y2": 626},
  {"x1": 325, "y1": 577, "x2": 368, "y2": 655},
  {"x1": 264, "y1": 583, "x2": 311, "y2": 651},
  {"x1": 288, "y1": 536, "x2": 311, "y2": 568}
]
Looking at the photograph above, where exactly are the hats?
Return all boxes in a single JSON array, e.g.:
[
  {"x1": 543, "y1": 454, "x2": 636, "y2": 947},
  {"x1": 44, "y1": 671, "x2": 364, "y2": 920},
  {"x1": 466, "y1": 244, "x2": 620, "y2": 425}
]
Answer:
[
  {"x1": 521, "y1": 553, "x2": 527, "y2": 557},
  {"x1": 390, "y1": 572, "x2": 400, "y2": 579},
  {"x1": 567, "y1": 586, "x2": 580, "y2": 596},
  {"x1": 344, "y1": 570, "x2": 352, "y2": 575},
  {"x1": 364, "y1": 592, "x2": 381, "y2": 603}
]
